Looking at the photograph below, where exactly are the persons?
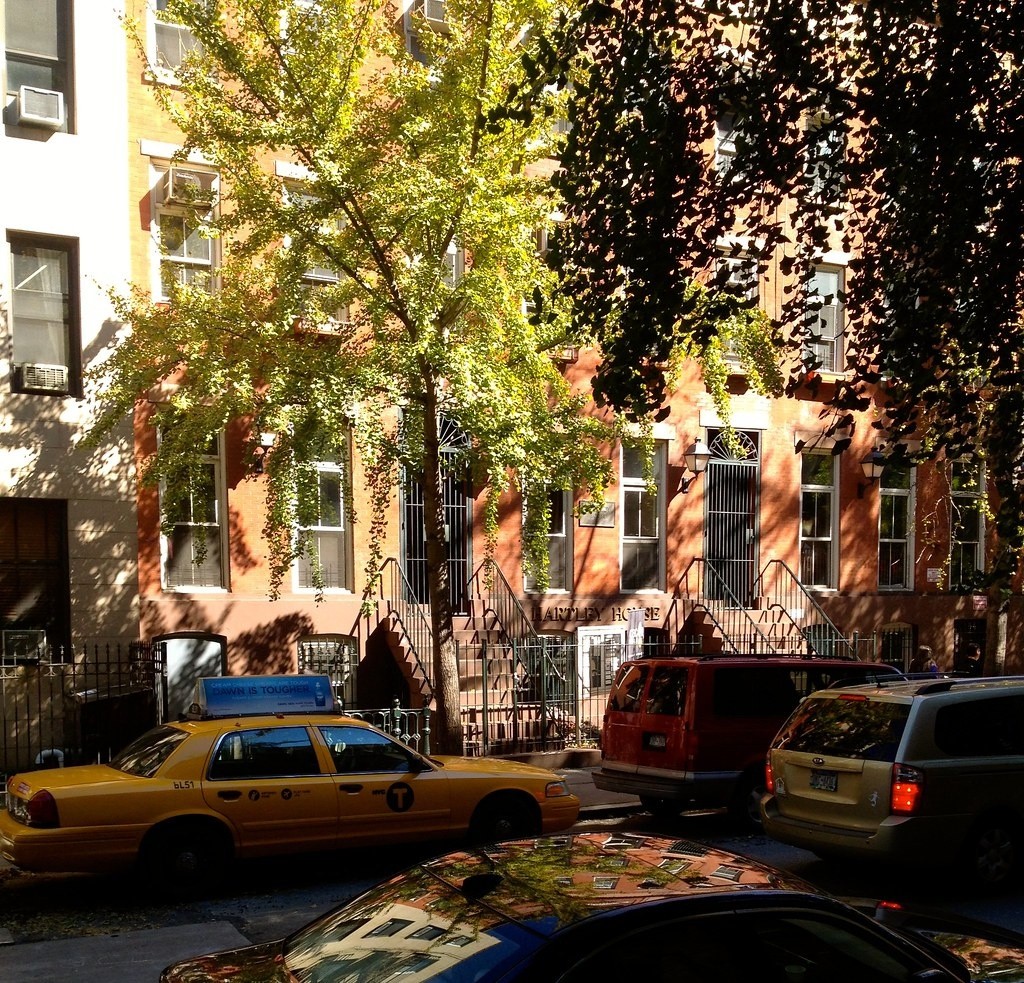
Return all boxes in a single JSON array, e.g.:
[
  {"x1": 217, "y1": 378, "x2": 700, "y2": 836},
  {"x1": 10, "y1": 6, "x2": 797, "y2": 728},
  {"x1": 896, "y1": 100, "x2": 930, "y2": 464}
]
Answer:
[
  {"x1": 907, "y1": 645, "x2": 943, "y2": 685},
  {"x1": 950, "y1": 643, "x2": 985, "y2": 678}
]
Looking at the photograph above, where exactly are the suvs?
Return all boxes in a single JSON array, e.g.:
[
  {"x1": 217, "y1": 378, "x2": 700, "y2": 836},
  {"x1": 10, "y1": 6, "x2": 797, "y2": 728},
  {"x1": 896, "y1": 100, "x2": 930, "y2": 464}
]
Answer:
[{"x1": 758, "y1": 667, "x2": 1024, "y2": 887}]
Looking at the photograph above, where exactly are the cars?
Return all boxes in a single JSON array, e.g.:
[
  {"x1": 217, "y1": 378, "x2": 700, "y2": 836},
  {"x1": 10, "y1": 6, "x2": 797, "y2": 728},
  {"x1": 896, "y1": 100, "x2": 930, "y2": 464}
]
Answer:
[
  {"x1": 159, "y1": 829, "x2": 1024, "y2": 983},
  {"x1": 0, "y1": 670, "x2": 582, "y2": 903}
]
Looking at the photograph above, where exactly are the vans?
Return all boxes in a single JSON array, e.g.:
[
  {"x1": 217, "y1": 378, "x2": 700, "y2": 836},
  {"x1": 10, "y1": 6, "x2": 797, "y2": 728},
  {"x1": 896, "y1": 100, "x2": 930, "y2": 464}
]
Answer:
[{"x1": 592, "y1": 650, "x2": 915, "y2": 824}]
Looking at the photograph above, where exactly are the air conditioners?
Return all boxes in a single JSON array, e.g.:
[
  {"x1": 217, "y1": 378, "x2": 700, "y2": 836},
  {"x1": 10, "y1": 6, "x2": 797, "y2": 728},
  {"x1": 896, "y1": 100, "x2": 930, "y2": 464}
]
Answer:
[
  {"x1": 164, "y1": 165, "x2": 217, "y2": 209},
  {"x1": 14, "y1": 361, "x2": 69, "y2": 395},
  {"x1": 14, "y1": 81, "x2": 67, "y2": 130},
  {"x1": 412, "y1": 2, "x2": 455, "y2": 35}
]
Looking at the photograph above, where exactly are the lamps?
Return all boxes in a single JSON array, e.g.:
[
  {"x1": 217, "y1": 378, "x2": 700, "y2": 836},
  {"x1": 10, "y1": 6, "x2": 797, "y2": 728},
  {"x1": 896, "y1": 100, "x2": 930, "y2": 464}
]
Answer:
[
  {"x1": 857, "y1": 445, "x2": 889, "y2": 500},
  {"x1": 246, "y1": 415, "x2": 281, "y2": 474},
  {"x1": 681, "y1": 438, "x2": 713, "y2": 495}
]
